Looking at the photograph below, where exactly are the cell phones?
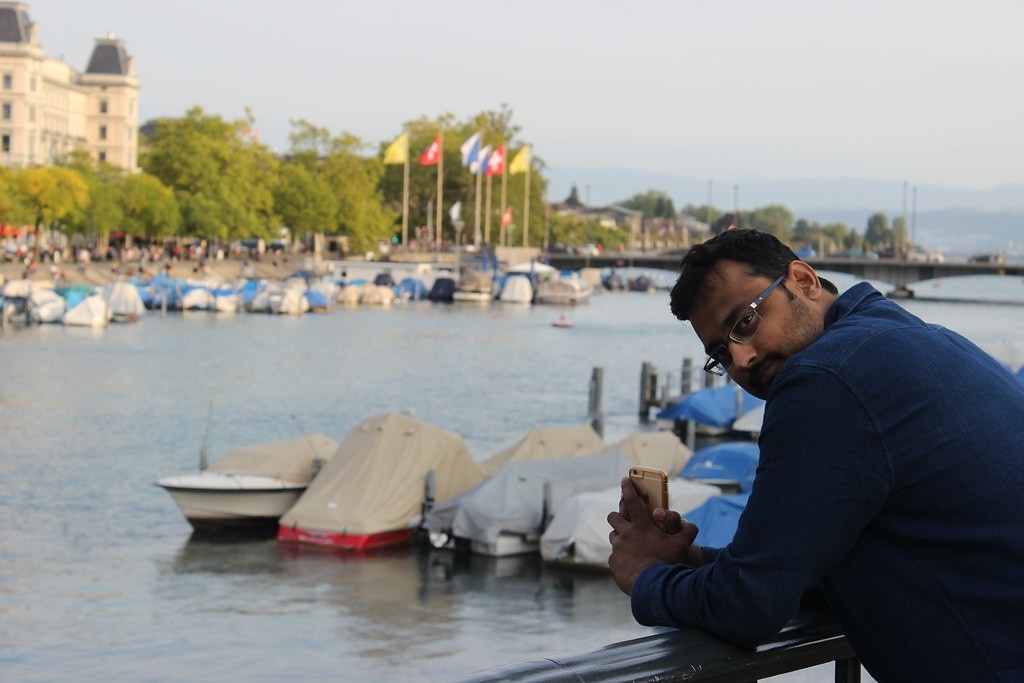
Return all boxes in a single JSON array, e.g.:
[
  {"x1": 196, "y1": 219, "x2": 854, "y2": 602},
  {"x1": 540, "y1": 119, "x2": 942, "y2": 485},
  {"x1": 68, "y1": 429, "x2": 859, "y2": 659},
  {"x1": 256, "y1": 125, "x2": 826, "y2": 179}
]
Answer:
[{"x1": 628, "y1": 467, "x2": 670, "y2": 530}]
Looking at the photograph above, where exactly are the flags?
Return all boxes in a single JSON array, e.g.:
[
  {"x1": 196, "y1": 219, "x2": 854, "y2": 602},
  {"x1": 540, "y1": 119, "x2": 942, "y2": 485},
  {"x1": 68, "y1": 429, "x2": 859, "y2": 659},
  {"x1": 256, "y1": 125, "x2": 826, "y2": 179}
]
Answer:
[
  {"x1": 419, "y1": 134, "x2": 445, "y2": 167},
  {"x1": 459, "y1": 132, "x2": 507, "y2": 177},
  {"x1": 382, "y1": 132, "x2": 410, "y2": 165},
  {"x1": 508, "y1": 145, "x2": 530, "y2": 175}
]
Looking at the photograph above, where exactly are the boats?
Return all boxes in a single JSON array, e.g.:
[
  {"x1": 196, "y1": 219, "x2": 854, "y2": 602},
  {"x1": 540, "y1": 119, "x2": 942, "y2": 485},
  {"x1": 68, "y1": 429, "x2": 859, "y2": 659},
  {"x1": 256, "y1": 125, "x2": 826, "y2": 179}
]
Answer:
[
  {"x1": 679, "y1": 488, "x2": 751, "y2": 549},
  {"x1": 404, "y1": 440, "x2": 638, "y2": 558},
  {"x1": 275, "y1": 407, "x2": 488, "y2": 551},
  {"x1": 477, "y1": 381, "x2": 766, "y2": 497},
  {"x1": 0, "y1": 248, "x2": 597, "y2": 332},
  {"x1": 151, "y1": 398, "x2": 341, "y2": 534},
  {"x1": 534, "y1": 471, "x2": 723, "y2": 570}
]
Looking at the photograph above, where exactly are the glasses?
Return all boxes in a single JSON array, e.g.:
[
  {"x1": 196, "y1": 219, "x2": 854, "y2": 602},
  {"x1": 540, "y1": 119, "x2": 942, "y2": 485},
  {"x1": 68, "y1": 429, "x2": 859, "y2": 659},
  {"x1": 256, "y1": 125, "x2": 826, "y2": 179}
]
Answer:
[{"x1": 704, "y1": 274, "x2": 787, "y2": 376}]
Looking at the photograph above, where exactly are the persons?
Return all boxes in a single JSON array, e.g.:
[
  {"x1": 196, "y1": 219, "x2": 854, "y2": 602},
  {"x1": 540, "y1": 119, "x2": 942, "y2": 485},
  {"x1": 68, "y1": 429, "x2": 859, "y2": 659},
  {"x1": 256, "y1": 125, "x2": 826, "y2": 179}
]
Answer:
[
  {"x1": 3, "y1": 235, "x2": 308, "y2": 283},
  {"x1": 606, "y1": 228, "x2": 1024, "y2": 683},
  {"x1": 361, "y1": 237, "x2": 455, "y2": 265}
]
